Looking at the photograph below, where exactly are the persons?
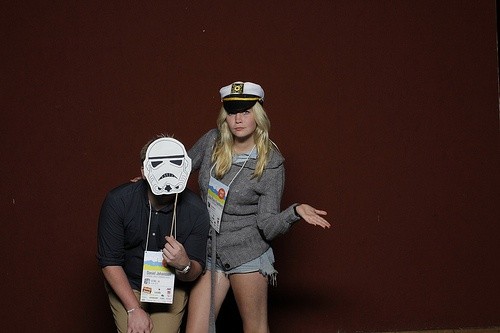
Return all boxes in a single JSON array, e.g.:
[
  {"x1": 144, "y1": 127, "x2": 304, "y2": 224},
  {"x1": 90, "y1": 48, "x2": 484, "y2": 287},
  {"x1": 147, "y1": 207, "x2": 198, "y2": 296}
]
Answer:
[
  {"x1": 96, "y1": 138, "x2": 211, "y2": 333},
  {"x1": 129, "y1": 81, "x2": 331, "y2": 333}
]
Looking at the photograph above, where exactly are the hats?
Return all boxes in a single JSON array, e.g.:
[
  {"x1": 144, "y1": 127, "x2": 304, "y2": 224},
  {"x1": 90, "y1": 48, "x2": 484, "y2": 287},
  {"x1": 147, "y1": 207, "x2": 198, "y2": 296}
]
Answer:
[{"x1": 219, "y1": 81, "x2": 265, "y2": 115}]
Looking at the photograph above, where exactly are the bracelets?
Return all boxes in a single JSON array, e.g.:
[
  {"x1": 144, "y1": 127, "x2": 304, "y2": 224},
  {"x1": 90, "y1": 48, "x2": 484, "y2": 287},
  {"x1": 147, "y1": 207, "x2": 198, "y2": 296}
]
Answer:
[{"x1": 127, "y1": 304, "x2": 141, "y2": 314}]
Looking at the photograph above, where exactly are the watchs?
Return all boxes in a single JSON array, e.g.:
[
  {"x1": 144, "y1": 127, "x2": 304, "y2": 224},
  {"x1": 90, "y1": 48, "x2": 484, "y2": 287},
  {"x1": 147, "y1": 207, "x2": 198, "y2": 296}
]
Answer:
[{"x1": 175, "y1": 261, "x2": 192, "y2": 280}]
